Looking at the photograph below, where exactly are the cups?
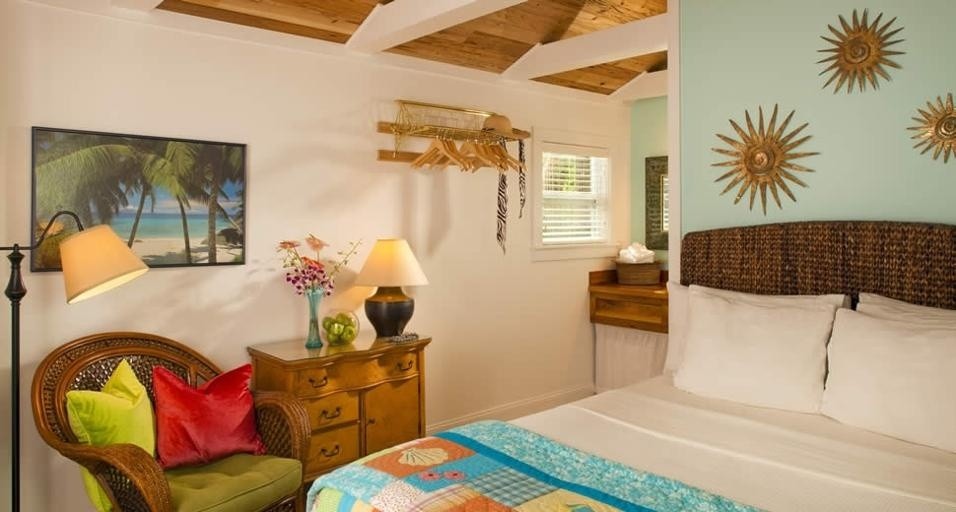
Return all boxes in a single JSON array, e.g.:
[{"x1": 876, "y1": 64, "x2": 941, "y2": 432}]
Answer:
[{"x1": 320, "y1": 309, "x2": 360, "y2": 346}]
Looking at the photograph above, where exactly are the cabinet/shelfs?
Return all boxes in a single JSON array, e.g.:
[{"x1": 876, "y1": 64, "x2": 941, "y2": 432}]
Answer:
[
  {"x1": 247, "y1": 332, "x2": 433, "y2": 512},
  {"x1": 593, "y1": 323, "x2": 668, "y2": 396}
]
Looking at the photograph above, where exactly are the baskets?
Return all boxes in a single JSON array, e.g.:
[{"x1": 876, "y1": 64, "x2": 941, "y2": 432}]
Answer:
[{"x1": 613, "y1": 261, "x2": 663, "y2": 284}]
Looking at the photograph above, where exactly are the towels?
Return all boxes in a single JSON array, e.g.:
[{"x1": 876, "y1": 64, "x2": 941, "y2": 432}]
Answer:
[{"x1": 618, "y1": 241, "x2": 653, "y2": 263}]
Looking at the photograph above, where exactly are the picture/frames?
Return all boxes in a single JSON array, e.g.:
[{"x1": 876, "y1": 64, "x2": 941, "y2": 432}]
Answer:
[{"x1": 28, "y1": 125, "x2": 249, "y2": 268}]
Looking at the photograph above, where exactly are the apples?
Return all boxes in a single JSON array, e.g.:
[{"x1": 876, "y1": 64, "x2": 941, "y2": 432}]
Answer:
[{"x1": 323, "y1": 313, "x2": 358, "y2": 347}]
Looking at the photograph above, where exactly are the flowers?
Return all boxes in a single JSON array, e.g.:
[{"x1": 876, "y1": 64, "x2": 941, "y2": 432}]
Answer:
[{"x1": 280, "y1": 235, "x2": 363, "y2": 296}]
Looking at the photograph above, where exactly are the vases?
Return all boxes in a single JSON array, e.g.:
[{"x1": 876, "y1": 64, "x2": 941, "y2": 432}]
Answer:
[{"x1": 304, "y1": 288, "x2": 322, "y2": 349}]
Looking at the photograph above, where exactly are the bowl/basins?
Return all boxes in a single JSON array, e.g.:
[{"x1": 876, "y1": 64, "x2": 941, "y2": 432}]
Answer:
[{"x1": 611, "y1": 258, "x2": 665, "y2": 285}]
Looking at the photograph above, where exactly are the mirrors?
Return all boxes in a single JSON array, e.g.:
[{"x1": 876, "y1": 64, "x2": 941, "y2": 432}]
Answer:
[{"x1": 642, "y1": 153, "x2": 669, "y2": 250}]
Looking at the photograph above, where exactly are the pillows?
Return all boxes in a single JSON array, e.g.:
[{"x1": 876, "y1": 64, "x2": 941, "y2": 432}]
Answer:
[
  {"x1": 820, "y1": 306, "x2": 954, "y2": 452},
  {"x1": 59, "y1": 355, "x2": 159, "y2": 512},
  {"x1": 688, "y1": 281, "x2": 852, "y2": 309},
  {"x1": 148, "y1": 360, "x2": 267, "y2": 472},
  {"x1": 856, "y1": 290, "x2": 953, "y2": 319},
  {"x1": 661, "y1": 279, "x2": 836, "y2": 415}
]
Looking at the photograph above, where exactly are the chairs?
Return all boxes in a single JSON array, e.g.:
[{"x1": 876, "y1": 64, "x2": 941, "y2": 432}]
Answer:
[{"x1": 27, "y1": 322, "x2": 307, "y2": 512}]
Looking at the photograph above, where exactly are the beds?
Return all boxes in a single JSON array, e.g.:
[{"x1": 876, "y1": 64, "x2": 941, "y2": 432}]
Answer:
[{"x1": 297, "y1": 216, "x2": 953, "y2": 512}]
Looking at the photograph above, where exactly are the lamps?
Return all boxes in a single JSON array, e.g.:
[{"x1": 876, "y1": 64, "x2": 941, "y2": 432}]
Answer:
[
  {"x1": 0, "y1": 202, "x2": 151, "y2": 512},
  {"x1": 359, "y1": 237, "x2": 430, "y2": 340}
]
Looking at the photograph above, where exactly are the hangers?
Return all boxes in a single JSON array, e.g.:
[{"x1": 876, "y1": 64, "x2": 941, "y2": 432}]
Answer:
[{"x1": 410, "y1": 129, "x2": 527, "y2": 179}]
